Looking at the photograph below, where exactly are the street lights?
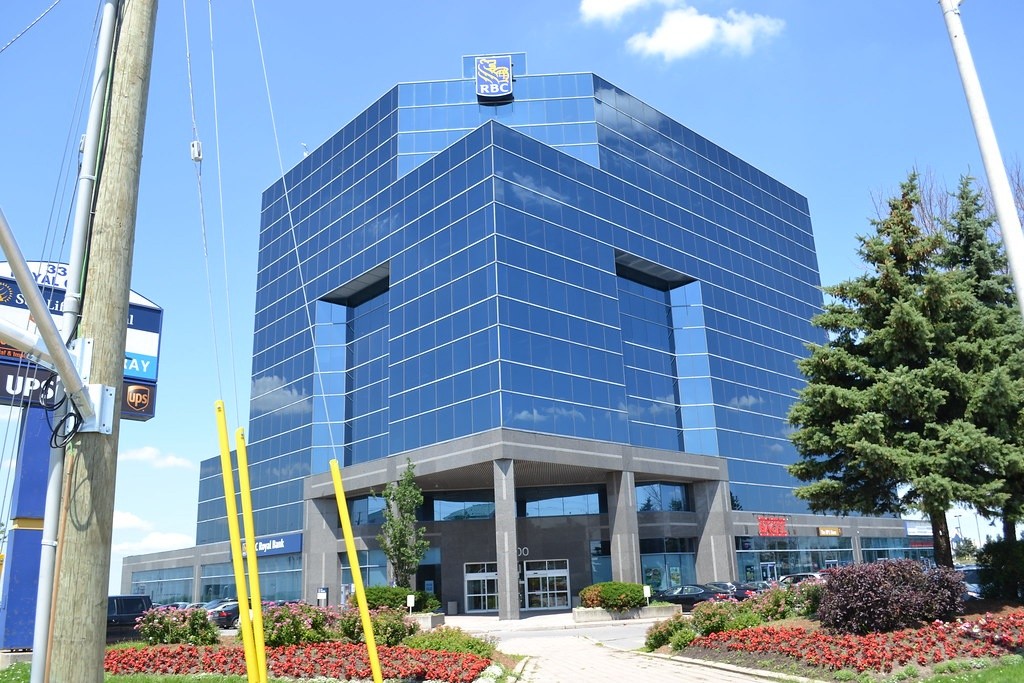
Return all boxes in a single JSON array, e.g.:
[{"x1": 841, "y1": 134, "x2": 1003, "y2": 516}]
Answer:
[
  {"x1": 954, "y1": 515, "x2": 966, "y2": 560},
  {"x1": 973, "y1": 512, "x2": 982, "y2": 551}
]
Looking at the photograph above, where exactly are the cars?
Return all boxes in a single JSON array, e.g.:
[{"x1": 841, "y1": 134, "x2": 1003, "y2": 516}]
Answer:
[
  {"x1": 954, "y1": 565, "x2": 990, "y2": 599},
  {"x1": 649, "y1": 583, "x2": 734, "y2": 612},
  {"x1": 746, "y1": 578, "x2": 787, "y2": 594},
  {"x1": 153, "y1": 597, "x2": 253, "y2": 629},
  {"x1": 704, "y1": 579, "x2": 760, "y2": 601}
]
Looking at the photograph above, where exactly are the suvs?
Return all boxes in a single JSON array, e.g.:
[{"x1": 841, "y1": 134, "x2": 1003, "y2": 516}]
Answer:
[
  {"x1": 780, "y1": 572, "x2": 837, "y2": 590},
  {"x1": 106, "y1": 595, "x2": 155, "y2": 642}
]
